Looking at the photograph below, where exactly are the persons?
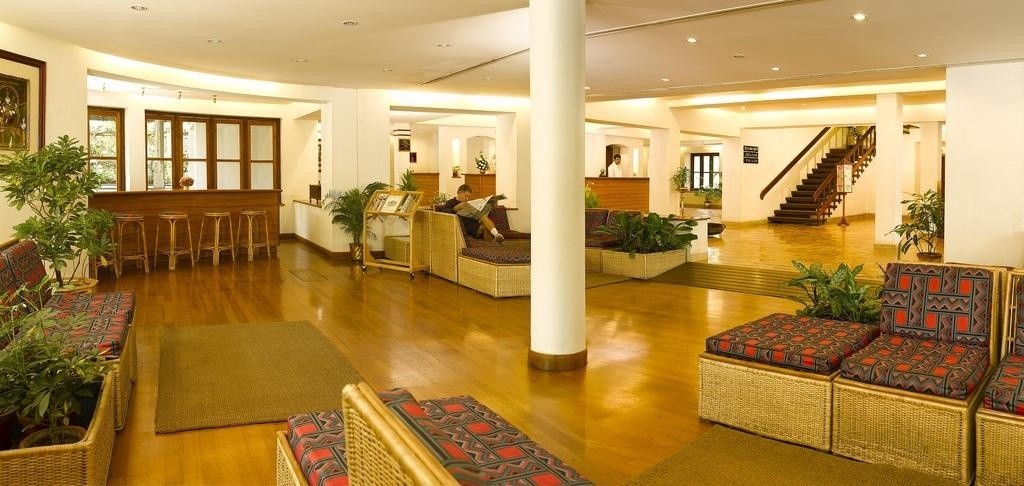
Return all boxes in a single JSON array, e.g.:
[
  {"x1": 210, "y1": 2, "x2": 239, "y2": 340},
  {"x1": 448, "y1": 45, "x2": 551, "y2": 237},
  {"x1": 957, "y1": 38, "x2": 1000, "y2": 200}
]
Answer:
[
  {"x1": 607, "y1": 154, "x2": 623, "y2": 177},
  {"x1": 439, "y1": 183, "x2": 506, "y2": 244}
]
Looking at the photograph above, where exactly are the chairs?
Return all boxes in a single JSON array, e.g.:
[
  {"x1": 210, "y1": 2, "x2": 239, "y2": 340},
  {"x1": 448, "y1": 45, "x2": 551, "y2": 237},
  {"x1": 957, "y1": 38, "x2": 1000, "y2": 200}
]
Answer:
[
  {"x1": 830, "y1": 261, "x2": 1001, "y2": 482},
  {"x1": 411, "y1": 200, "x2": 648, "y2": 300},
  {"x1": 272, "y1": 375, "x2": 599, "y2": 486},
  {"x1": 972, "y1": 264, "x2": 1024, "y2": 484},
  {"x1": 0, "y1": 235, "x2": 137, "y2": 433}
]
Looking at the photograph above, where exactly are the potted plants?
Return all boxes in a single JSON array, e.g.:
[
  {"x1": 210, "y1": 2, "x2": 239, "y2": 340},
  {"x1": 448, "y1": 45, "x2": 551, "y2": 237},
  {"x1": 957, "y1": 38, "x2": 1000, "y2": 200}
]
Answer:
[
  {"x1": 695, "y1": 172, "x2": 722, "y2": 209},
  {"x1": 1, "y1": 129, "x2": 120, "y2": 450},
  {"x1": 670, "y1": 163, "x2": 693, "y2": 193},
  {"x1": 880, "y1": 188, "x2": 945, "y2": 263},
  {"x1": 323, "y1": 179, "x2": 394, "y2": 262}
]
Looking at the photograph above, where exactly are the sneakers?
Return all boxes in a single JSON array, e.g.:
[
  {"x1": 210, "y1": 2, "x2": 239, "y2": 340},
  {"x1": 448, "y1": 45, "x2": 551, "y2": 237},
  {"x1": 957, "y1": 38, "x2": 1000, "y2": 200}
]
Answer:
[{"x1": 494, "y1": 234, "x2": 504, "y2": 244}]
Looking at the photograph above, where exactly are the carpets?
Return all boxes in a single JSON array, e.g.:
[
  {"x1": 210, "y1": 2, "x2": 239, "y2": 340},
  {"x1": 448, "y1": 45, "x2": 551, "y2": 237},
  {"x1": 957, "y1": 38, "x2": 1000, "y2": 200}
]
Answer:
[
  {"x1": 622, "y1": 423, "x2": 973, "y2": 485},
  {"x1": 153, "y1": 318, "x2": 376, "y2": 435},
  {"x1": 650, "y1": 261, "x2": 883, "y2": 307}
]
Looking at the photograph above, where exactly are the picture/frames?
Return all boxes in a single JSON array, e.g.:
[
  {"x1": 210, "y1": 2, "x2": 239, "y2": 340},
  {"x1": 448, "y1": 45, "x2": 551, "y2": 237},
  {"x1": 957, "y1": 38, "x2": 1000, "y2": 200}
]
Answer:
[{"x1": 0, "y1": 49, "x2": 49, "y2": 179}]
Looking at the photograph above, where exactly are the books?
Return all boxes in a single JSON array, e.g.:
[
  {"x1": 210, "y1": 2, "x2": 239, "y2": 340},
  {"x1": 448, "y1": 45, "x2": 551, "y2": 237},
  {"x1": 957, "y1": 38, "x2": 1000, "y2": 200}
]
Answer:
[{"x1": 380, "y1": 195, "x2": 403, "y2": 213}]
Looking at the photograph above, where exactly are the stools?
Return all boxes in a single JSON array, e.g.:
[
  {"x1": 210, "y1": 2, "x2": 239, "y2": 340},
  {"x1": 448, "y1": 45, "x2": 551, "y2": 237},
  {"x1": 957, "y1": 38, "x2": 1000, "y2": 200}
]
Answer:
[
  {"x1": 697, "y1": 312, "x2": 880, "y2": 454},
  {"x1": 89, "y1": 208, "x2": 271, "y2": 279}
]
